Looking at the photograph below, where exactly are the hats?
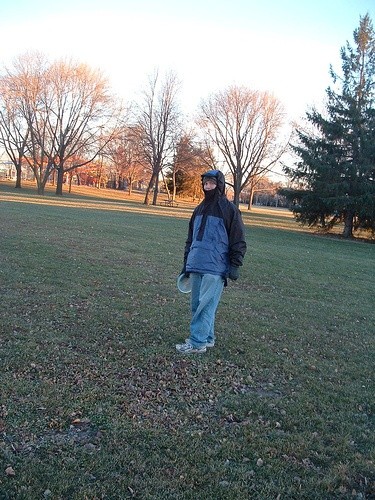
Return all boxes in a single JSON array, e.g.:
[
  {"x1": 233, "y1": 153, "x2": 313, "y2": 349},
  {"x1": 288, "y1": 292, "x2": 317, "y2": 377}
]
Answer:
[{"x1": 203, "y1": 176, "x2": 217, "y2": 185}]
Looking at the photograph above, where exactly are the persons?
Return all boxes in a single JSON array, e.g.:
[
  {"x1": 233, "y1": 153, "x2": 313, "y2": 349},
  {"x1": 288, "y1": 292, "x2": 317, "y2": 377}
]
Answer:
[{"x1": 175, "y1": 169, "x2": 247, "y2": 355}]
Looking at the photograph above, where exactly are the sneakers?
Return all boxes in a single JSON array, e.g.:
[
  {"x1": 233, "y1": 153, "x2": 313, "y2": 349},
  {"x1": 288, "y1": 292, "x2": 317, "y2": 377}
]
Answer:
[
  {"x1": 185, "y1": 339, "x2": 214, "y2": 347},
  {"x1": 176, "y1": 342, "x2": 207, "y2": 354}
]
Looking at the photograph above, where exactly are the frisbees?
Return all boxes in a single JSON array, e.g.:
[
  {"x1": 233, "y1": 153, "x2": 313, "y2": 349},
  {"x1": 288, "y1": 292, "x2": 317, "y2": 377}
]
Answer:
[{"x1": 176, "y1": 272, "x2": 193, "y2": 293}]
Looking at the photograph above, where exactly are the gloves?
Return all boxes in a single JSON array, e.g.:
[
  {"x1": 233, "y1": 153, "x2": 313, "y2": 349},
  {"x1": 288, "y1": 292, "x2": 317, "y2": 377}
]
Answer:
[
  {"x1": 226, "y1": 265, "x2": 239, "y2": 280},
  {"x1": 179, "y1": 266, "x2": 189, "y2": 281}
]
